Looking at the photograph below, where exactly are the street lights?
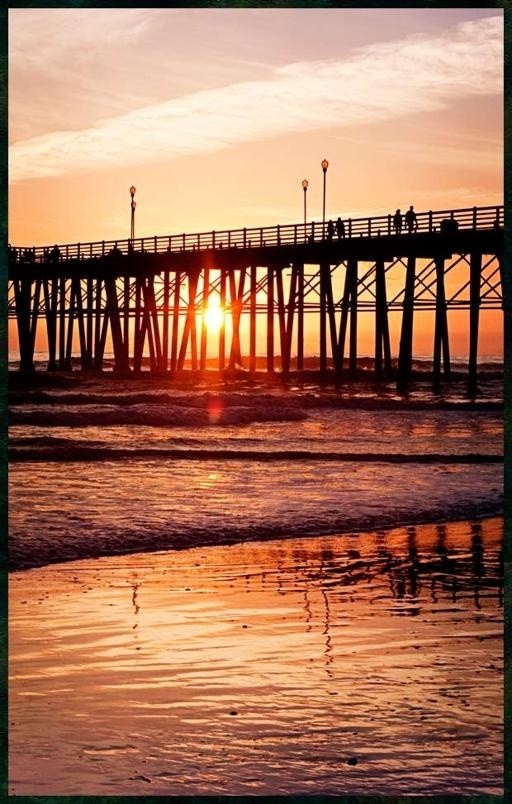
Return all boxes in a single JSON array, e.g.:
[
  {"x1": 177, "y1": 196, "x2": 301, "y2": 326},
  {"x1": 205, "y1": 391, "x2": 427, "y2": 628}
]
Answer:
[
  {"x1": 130, "y1": 185, "x2": 138, "y2": 249},
  {"x1": 302, "y1": 179, "x2": 308, "y2": 243},
  {"x1": 321, "y1": 158, "x2": 328, "y2": 238}
]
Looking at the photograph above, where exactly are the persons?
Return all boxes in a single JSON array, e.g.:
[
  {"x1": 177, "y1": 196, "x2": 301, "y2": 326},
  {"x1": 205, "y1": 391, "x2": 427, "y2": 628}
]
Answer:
[
  {"x1": 22, "y1": 247, "x2": 36, "y2": 265},
  {"x1": 9, "y1": 247, "x2": 18, "y2": 263},
  {"x1": 404, "y1": 206, "x2": 419, "y2": 234},
  {"x1": 113, "y1": 245, "x2": 119, "y2": 257},
  {"x1": 335, "y1": 217, "x2": 345, "y2": 239},
  {"x1": 394, "y1": 209, "x2": 403, "y2": 237},
  {"x1": 44, "y1": 245, "x2": 60, "y2": 263},
  {"x1": 328, "y1": 219, "x2": 335, "y2": 241}
]
